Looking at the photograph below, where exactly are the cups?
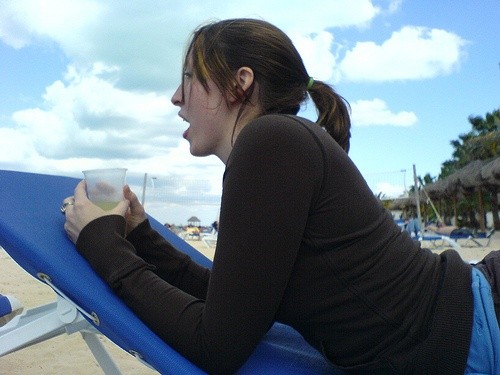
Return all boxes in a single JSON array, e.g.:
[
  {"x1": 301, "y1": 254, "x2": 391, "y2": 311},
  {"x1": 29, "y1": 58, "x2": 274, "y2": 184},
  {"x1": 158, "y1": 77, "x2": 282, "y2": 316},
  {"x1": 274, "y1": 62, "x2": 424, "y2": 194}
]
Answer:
[{"x1": 82, "y1": 167, "x2": 129, "y2": 237}]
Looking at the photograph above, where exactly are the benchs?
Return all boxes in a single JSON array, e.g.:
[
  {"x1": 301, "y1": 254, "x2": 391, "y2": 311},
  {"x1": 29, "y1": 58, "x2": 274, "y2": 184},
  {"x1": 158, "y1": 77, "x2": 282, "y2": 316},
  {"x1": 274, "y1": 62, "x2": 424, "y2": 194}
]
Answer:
[
  {"x1": 422, "y1": 227, "x2": 494, "y2": 247},
  {"x1": 0, "y1": 171, "x2": 342, "y2": 374}
]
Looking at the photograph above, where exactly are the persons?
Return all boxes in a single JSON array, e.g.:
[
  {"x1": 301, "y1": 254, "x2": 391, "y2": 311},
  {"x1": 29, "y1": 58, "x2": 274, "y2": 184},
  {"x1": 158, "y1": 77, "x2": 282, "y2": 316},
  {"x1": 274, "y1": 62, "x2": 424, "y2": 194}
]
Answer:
[
  {"x1": 427, "y1": 221, "x2": 495, "y2": 237},
  {"x1": 166, "y1": 219, "x2": 219, "y2": 239},
  {"x1": 59, "y1": 12, "x2": 500, "y2": 375}
]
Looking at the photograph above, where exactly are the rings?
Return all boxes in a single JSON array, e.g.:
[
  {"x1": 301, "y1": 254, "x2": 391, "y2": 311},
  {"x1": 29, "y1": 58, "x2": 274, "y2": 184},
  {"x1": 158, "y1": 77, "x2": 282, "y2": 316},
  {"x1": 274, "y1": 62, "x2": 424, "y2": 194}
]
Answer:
[{"x1": 61, "y1": 202, "x2": 73, "y2": 214}]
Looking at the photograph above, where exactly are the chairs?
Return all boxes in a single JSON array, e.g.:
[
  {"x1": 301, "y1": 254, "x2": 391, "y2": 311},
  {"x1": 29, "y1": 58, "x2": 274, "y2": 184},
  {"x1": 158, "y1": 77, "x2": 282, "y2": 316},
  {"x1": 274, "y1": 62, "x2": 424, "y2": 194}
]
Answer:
[{"x1": 202, "y1": 235, "x2": 217, "y2": 247}]
